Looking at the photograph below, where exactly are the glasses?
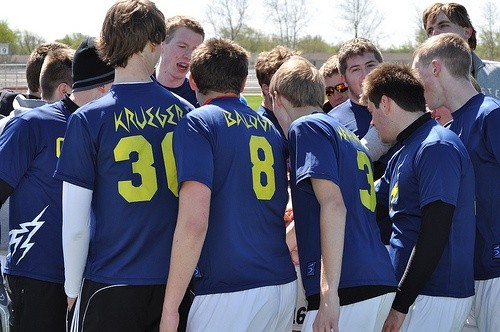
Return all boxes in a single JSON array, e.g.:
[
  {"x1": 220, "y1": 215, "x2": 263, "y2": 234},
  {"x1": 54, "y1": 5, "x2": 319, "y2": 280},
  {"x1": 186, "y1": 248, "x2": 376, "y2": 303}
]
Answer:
[{"x1": 326, "y1": 82, "x2": 349, "y2": 96}]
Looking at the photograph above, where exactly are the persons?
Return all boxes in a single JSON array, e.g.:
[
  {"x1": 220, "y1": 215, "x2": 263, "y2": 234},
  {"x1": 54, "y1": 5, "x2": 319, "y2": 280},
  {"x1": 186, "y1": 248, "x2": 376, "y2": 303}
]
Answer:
[
  {"x1": 357, "y1": 60, "x2": 477, "y2": 332},
  {"x1": 269, "y1": 56, "x2": 400, "y2": 332},
  {"x1": 151, "y1": 2, "x2": 500, "y2": 265},
  {"x1": 0, "y1": 37, "x2": 114, "y2": 332},
  {"x1": 52, "y1": 0, "x2": 199, "y2": 332},
  {"x1": 158, "y1": 37, "x2": 299, "y2": 332},
  {"x1": 409, "y1": 32, "x2": 500, "y2": 331},
  {"x1": 0, "y1": 42, "x2": 76, "y2": 281}
]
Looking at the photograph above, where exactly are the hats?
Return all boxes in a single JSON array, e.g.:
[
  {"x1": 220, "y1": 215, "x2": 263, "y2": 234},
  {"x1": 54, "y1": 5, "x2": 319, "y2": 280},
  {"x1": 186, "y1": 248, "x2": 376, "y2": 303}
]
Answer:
[{"x1": 72, "y1": 37, "x2": 115, "y2": 93}]
